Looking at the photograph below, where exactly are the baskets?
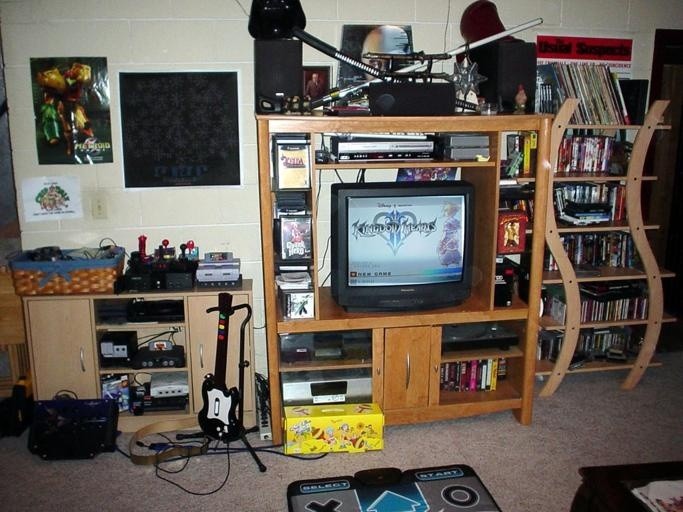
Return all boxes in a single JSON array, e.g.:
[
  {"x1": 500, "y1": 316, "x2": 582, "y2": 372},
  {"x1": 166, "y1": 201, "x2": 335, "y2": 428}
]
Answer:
[{"x1": 10, "y1": 247, "x2": 124, "y2": 297}]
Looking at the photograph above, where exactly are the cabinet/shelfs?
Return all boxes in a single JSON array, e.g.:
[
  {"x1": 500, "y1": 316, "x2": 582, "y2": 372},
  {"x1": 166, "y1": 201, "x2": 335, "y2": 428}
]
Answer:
[
  {"x1": 528, "y1": 99, "x2": 678, "y2": 400},
  {"x1": 253, "y1": 110, "x2": 555, "y2": 449},
  {"x1": 22, "y1": 280, "x2": 253, "y2": 435}
]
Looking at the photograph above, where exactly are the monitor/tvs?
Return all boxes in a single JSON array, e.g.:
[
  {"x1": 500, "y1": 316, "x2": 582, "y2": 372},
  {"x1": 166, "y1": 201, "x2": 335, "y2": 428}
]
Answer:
[{"x1": 330, "y1": 181, "x2": 474, "y2": 314}]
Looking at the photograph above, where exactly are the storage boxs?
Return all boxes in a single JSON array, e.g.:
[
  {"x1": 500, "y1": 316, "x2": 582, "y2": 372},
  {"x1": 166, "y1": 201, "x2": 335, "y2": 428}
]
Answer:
[{"x1": 280, "y1": 401, "x2": 382, "y2": 453}]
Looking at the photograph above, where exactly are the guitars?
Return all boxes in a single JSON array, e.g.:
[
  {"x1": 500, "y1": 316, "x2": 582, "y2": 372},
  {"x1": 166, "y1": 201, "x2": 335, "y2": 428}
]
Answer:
[{"x1": 199, "y1": 292, "x2": 238, "y2": 442}]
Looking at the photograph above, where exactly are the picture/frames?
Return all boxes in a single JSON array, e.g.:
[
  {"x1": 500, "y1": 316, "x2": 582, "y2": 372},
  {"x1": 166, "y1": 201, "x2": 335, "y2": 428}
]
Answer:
[{"x1": 301, "y1": 61, "x2": 339, "y2": 109}]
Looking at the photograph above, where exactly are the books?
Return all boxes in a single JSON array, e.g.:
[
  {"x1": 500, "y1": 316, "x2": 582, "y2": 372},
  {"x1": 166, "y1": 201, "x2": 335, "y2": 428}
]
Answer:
[
  {"x1": 439, "y1": 358, "x2": 506, "y2": 394},
  {"x1": 494, "y1": 129, "x2": 533, "y2": 311},
  {"x1": 535, "y1": 60, "x2": 651, "y2": 369}
]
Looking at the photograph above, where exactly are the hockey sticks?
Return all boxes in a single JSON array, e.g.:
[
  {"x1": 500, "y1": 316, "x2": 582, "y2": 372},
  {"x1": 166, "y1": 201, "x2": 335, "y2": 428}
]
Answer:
[{"x1": 260, "y1": 17, "x2": 542, "y2": 115}]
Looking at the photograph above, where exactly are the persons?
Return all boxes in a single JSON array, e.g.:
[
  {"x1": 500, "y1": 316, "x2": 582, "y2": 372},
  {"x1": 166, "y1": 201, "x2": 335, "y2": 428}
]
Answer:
[{"x1": 306, "y1": 74, "x2": 325, "y2": 98}]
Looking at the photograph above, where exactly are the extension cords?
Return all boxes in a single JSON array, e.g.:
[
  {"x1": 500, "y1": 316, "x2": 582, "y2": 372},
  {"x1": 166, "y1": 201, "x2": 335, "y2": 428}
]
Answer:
[{"x1": 256, "y1": 395, "x2": 271, "y2": 441}]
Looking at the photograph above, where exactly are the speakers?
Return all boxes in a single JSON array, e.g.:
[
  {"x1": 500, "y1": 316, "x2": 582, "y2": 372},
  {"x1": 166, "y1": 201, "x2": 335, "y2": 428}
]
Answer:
[
  {"x1": 465, "y1": 40, "x2": 540, "y2": 116},
  {"x1": 369, "y1": 81, "x2": 456, "y2": 117},
  {"x1": 254, "y1": 38, "x2": 305, "y2": 113}
]
